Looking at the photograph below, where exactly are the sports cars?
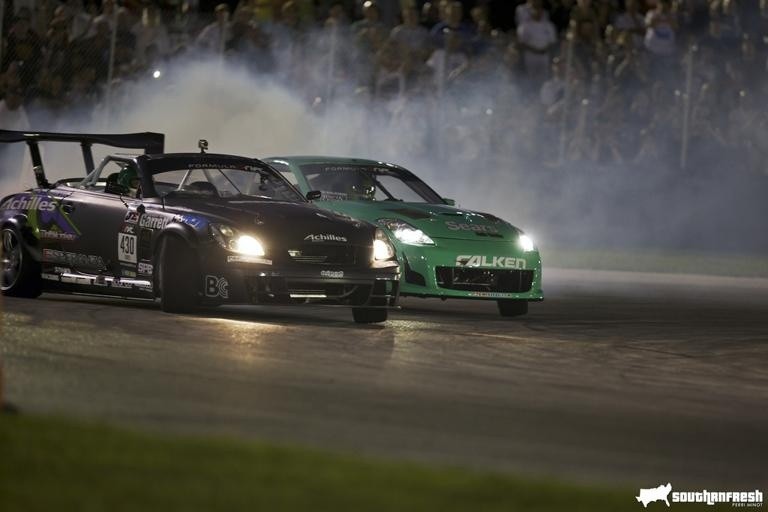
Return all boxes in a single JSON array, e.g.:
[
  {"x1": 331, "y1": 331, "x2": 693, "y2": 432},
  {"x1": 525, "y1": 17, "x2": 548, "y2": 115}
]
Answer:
[
  {"x1": 206, "y1": 156, "x2": 544, "y2": 317},
  {"x1": 2, "y1": 129, "x2": 401, "y2": 322}
]
